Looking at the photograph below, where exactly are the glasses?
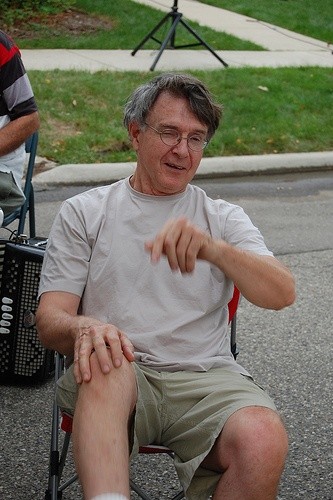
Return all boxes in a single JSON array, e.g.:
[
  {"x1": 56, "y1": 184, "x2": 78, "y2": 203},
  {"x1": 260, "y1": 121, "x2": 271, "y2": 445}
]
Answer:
[{"x1": 142, "y1": 121, "x2": 210, "y2": 153}]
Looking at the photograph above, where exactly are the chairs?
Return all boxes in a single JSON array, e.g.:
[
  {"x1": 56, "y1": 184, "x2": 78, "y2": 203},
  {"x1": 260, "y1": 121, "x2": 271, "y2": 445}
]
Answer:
[
  {"x1": 44, "y1": 283, "x2": 240, "y2": 500},
  {"x1": 2, "y1": 130, "x2": 39, "y2": 240}
]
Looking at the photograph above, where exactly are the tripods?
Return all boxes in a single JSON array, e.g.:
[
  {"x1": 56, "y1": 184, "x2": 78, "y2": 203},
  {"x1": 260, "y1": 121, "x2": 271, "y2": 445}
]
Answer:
[{"x1": 131, "y1": 0, "x2": 229, "y2": 72}]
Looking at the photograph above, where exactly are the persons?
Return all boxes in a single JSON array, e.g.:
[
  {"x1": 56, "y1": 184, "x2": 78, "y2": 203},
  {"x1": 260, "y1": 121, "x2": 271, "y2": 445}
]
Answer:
[
  {"x1": 0, "y1": 29, "x2": 41, "y2": 214},
  {"x1": 36, "y1": 73, "x2": 297, "y2": 500}
]
